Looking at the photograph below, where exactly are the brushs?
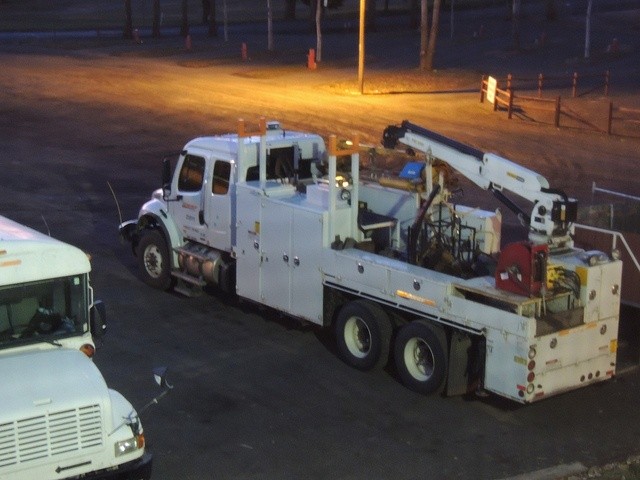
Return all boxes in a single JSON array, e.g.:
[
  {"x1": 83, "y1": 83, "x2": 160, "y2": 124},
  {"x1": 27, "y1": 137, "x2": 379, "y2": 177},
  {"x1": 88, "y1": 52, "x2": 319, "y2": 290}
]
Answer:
[{"x1": 1, "y1": 331, "x2": 86, "y2": 347}]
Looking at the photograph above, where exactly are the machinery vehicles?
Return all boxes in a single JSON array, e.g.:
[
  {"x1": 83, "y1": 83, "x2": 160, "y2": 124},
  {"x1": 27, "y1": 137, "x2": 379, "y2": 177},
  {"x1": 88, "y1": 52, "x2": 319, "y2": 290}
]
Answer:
[
  {"x1": 0, "y1": 214, "x2": 178, "y2": 480},
  {"x1": 105, "y1": 116, "x2": 624, "y2": 404}
]
